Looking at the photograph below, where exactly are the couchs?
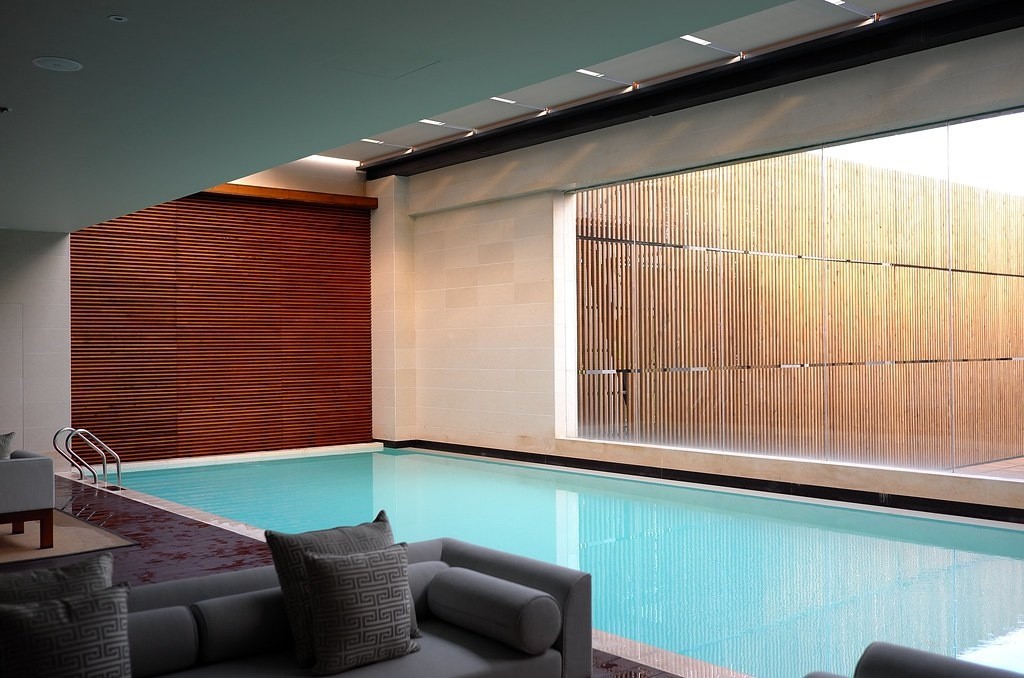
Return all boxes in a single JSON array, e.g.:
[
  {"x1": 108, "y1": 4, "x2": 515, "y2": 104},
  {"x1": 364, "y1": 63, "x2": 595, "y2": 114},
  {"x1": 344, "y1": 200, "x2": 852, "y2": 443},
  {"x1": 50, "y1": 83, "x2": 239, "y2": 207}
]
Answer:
[
  {"x1": 0, "y1": 449, "x2": 55, "y2": 549},
  {"x1": 126, "y1": 537, "x2": 593, "y2": 678}
]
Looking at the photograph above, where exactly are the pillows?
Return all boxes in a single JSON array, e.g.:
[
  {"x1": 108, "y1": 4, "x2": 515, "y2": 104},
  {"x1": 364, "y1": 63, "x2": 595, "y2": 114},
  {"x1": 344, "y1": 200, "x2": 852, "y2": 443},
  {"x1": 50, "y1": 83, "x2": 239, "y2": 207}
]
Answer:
[
  {"x1": 0, "y1": 551, "x2": 113, "y2": 603},
  {"x1": 0, "y1": 432, "x2": 16, "y2": 460},
  {"x1": 0, "y1": 581, "x2": 133, "y2": 678},
  {"x1": 265, "y1": 510, "x2": 423, "y2": 669},
  {"x1": 301, "y1": 543, "x2": 420, "y2": 678}
]
